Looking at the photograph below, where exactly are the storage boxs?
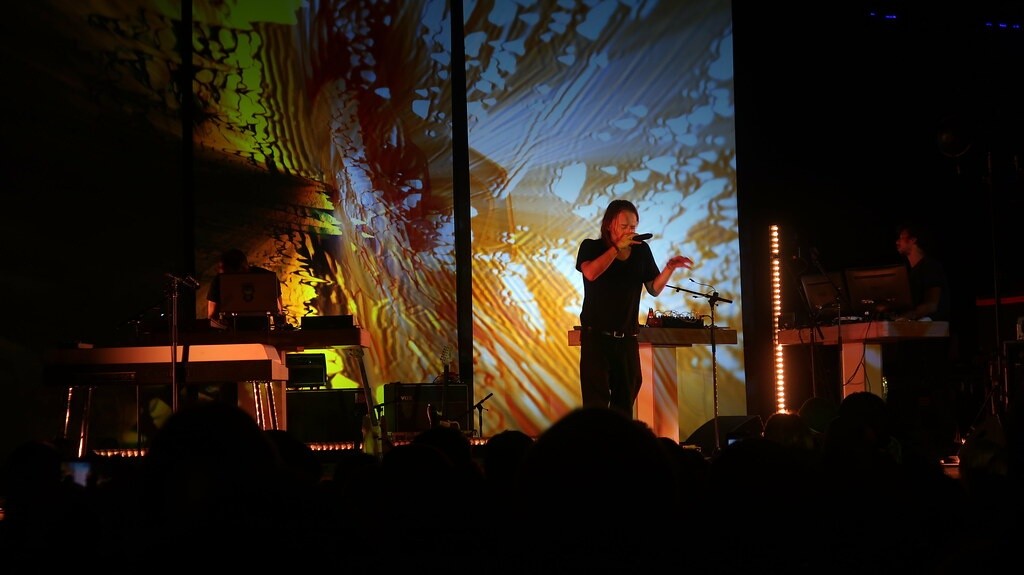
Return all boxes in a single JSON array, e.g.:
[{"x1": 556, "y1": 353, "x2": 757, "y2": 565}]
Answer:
[{"x1": 286, "y1": 354, "x2": 327, "y2": 387}]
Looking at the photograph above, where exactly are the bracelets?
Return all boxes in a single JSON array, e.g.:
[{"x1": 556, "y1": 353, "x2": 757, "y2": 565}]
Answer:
[
  {"x1": 668, "y1": 264, "x2": 674, "y2": 270},
  {"x1": 614, "y1": 245, "x2": 619, "y2": 253}
]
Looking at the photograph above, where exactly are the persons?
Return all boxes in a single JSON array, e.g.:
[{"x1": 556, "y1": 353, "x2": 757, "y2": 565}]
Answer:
[
  {"x1": 576, "y1": 200, "x2": 694, "y2": 420},
  {"x1": 1, "y1": 395, "x2": 1024, "y2": 575},
  {"x1": 896, "y1": 224, "x2": 949, "y2": 322}
]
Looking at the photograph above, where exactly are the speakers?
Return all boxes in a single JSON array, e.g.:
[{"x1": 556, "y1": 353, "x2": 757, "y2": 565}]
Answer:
[
  {"x1": 685, "y1": 415, "x2": 765, "y2": 459},
  {"x1": 384, "y1": 384, "x2": 470, "y2": 436},
  {"x1": 962, "y1": 412, "x2": 1024, "y2": 476},
  {"x1": 286, "y1": 388, "x2": 370, "y2": 461}
]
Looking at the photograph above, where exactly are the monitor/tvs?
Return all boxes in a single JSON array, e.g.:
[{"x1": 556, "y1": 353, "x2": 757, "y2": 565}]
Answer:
[{"x1": 801, "y1": 263, "x2": 913, "y2": 324}]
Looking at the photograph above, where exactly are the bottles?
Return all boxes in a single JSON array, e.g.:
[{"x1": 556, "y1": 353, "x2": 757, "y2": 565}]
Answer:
[{"x1": 648, "y1": 308, "x2": 654, "y2": 318}]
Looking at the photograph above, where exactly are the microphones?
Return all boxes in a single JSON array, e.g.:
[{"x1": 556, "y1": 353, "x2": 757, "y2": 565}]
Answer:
[
  {"x1": 632, "y1": 233, "x2": 653, "y2": 241},
  {"x1": 189, "y1": 275, "x2": 200, "y2": 287}
]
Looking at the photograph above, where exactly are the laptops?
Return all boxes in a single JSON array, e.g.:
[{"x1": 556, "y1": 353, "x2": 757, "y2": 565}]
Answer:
[{"x1": 219, "y1": 274, "x2": 278, "y2": 317}]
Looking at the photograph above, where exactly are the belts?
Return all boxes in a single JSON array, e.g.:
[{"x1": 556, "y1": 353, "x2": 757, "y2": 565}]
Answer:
[{"x1": 588, "y1": 327, "x2": 639, "y2": 338}]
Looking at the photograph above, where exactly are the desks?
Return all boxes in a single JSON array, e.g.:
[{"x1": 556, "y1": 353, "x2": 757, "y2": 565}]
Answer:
[
  {"x1": 777, "y1": 321, "x2": 949, "y2": 399},
  {"x1": 151, "y1": 328, "x2": 377, "y2": 431},
  {"x1": 568, "y1": 329, "x2": 738, "y2": 445}
]
